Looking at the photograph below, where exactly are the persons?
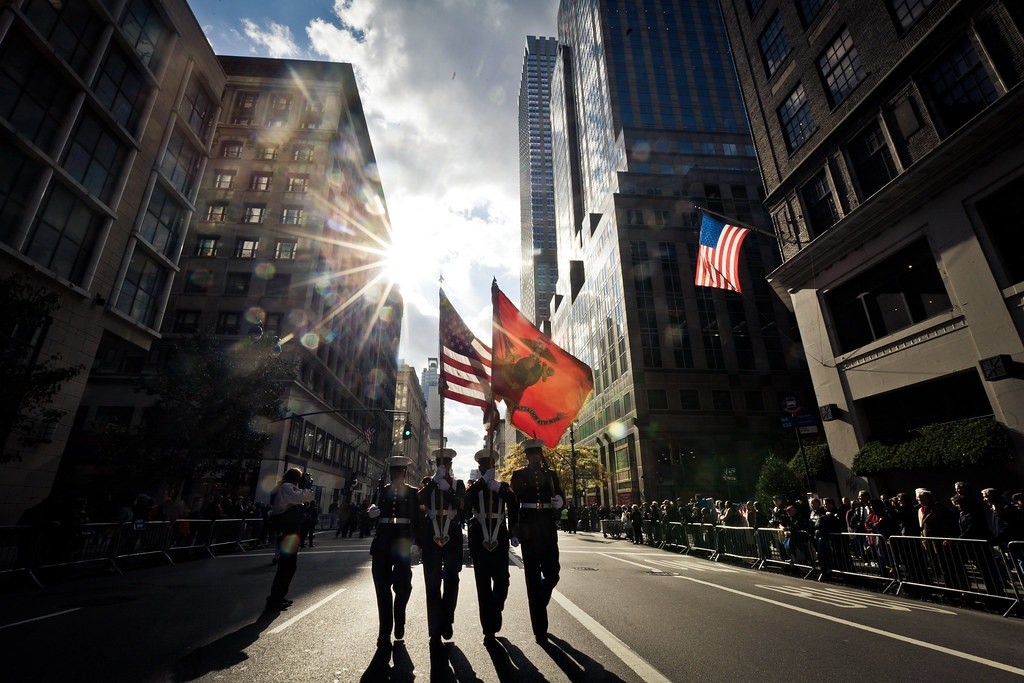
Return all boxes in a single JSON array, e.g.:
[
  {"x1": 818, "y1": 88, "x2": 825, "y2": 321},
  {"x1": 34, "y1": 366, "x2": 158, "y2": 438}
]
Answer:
[
  {"x1": 89, "y1": 492, "x2": 112, "y2": 546},
  {"x1": 367, "y1": 456, "x2": 420, "y2": 644},
  {"x1": 15, "y1": 491, "x2": 88, "y2": 566},
  {"x1": 328, "y1": 498, "x2": 372, "y2": 538},
  {"x1": 509, "y1": 439, "x2": 567, "y2": 642},
  {"x1": 263, "y1": 469, "x2": 319, "y2": 613},
  {"x1": 465, "y1": 448, "x2": 516, "y2": 643},
  {"x1": 560, "y1": 481, "x2": 1024, "y2": 614},
  {"x1": 105, "y1": 494, "x2": 272, "y2": 571},
  {"x1": 416, "y1": 448, "x2": 466, "y2": 647}
]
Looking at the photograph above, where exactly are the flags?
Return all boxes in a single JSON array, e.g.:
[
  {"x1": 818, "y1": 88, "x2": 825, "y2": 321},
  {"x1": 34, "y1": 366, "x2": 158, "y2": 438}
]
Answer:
[
  {"x1": 439, "y1": 288, "x2": 502, "y2": 439},
  {"x1": 491, "y1": 286, "x2": 594, "y2": 448},
  {"x1": 695, "y1": 212, "x2": 751, "y2": 293}
]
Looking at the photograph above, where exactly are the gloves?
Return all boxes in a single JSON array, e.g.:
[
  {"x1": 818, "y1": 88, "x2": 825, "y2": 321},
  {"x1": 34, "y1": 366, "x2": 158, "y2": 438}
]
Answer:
[
  {"x1": 511, "y1": 537, "x2": 519, "y2": 547},
  {"x1": 436, "y1": 478, "x2": 450, "y2": 491},
  {"x1": 368, "y1": 504, "x2": 381, "y2": 519},
  {"x1": 486, "y1": 478, "x2": 502, "y2": 492},
  {"x1": 552, "y1": 495, "x2": 563, "y2": 509},
  {"x1": 482, "y1": 469, "x2": 495, "y2": 486},
  {"x1": 433, "y1": 464, "x2": 446, "y2": 483}
]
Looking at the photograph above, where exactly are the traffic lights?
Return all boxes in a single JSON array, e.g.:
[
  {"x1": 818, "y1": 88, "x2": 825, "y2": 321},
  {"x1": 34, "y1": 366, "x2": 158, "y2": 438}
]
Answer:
[{"x1": 403, "y1": 419, "x2": 413, "y2": 440}]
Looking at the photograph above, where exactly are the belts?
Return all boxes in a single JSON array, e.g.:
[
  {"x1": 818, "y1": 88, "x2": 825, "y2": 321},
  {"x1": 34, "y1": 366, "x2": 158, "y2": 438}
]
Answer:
[
  {"x1": 426, "y1": 509, "x2": 456, "y2": 515},
  {"x1": 521, "y1": 502, "x2": 551, "y2": 509},
  {"x1": 379, "y1": 517, "x2": 411, "y2": 525}
]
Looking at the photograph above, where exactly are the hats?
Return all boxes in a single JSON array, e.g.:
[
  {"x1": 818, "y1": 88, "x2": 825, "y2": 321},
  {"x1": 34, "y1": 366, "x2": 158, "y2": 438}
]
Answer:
[
  {"x1": 385, "y1": 456, "x2": 412, "y2": 472},
  {"x1": 431, "y1": 448, "x2": 457, "y2": 465},
  {"x1": 474, "y1": 449, "x2": 500, "y2": 464},
  {"x1": 520, "y1": 440, "x2": 544, "y2": 455}
]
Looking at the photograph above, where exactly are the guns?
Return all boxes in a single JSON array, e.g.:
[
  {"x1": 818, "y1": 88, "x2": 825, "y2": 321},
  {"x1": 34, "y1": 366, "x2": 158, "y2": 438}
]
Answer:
[
  {"x1": 372, "y1": 440, "x2": 394, "y2": 526},
  {"x1": 533, "y1": 430, "x2": 559, "y2": 510}
]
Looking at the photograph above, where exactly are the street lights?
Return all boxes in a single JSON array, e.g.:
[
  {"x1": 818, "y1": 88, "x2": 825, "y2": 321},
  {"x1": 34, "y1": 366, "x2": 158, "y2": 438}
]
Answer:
[{"x1": 567, "y1": 416, "x2": 580, "y2": 530}]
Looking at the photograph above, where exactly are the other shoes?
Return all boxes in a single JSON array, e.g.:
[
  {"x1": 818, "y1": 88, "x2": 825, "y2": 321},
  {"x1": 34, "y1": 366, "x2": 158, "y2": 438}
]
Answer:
[
  {"x1": 394, "y1": 621, "x2": 404, "y2": 640},
  {"x1": 536, "y1": 632, "x2": 547, "y2": 644},
  {"x1": 377, "y1": 630, "x2": 390, "y2": 649},
  {"x1": 430, "y1": 635, "x2": 445, "y2": 650},
  {"x1": 495, "y1": 611, "x2": 502, "y2": 632},
  {"x1": 441, "y1": 623, "x2": 453, "y2": 640},
  {"x1": 543, "y1": 585, "x2": 550, "y2": 605},
  {"x1": 484, "y1": 634, "x2": 494, "y2": 646},
  {"x1": 266, "y1": 597, "x2": 294, "y2": 609}
]
